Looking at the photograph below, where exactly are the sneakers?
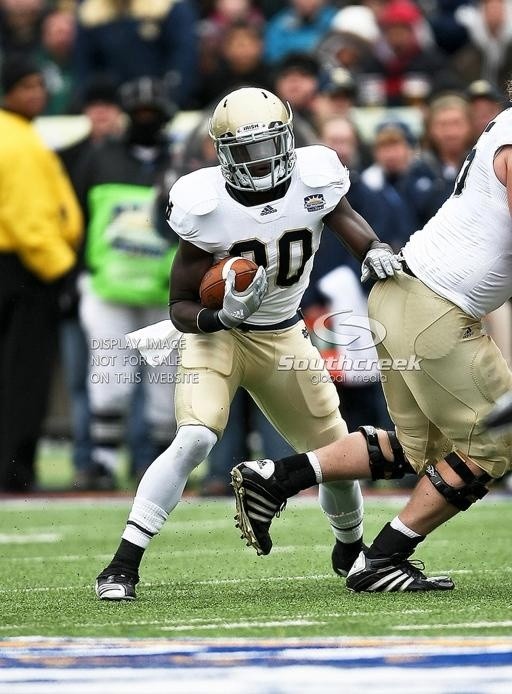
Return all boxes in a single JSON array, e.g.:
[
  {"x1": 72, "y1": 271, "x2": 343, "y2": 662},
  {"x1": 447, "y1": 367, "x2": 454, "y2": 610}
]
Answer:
[
  {"x1": 331, "y1": 545, "x2": 456, "y2": 593},
  {"x1": 95, "y1": 573, "x2": 137, "y2": 601},
  {"x1": 230, "y1": 458, "x2": 286, "y2": 556}
]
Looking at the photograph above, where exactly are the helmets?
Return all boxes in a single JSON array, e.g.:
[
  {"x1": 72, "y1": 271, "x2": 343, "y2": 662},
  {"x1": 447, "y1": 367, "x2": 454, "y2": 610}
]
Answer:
[{"x1": 207, "y1": 85, "x2": 297, "y2": 195}]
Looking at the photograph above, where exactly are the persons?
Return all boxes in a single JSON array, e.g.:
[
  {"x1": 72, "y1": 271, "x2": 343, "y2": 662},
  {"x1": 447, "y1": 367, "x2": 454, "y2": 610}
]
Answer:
[
  {"x1": 95, "y1": 88, "x2": 406, "y2": 599},
  {"x1": 229, "y1": 106, "x2": 512, "y2": 593}
]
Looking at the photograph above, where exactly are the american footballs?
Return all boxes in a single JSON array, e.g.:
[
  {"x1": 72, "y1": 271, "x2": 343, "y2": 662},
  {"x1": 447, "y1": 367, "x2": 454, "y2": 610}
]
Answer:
[{"x1": 198, "y1": 257, "x2": 259, "y2": 312}]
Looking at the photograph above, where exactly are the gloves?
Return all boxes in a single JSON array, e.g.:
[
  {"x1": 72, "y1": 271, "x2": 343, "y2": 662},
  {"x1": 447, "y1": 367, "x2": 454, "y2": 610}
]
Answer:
[
  {"x1": 359, "y1": 242, "x2": 405, "y2": 282},
  {"x1": 218, "y1": 264, "x2": 269, "y2": 330}
]
